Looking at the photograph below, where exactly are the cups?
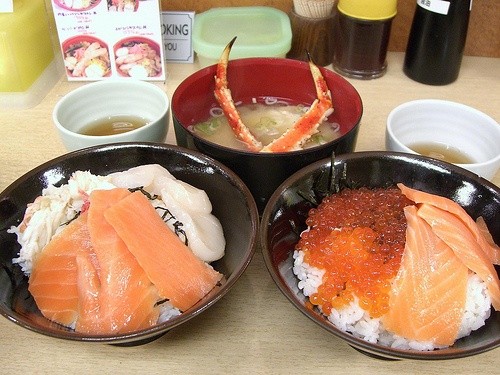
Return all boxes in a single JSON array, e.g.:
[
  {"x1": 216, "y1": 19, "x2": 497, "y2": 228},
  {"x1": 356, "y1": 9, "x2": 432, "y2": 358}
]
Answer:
[{"x1": 286, "y1": 7, "x2": 338, "y2": 67}]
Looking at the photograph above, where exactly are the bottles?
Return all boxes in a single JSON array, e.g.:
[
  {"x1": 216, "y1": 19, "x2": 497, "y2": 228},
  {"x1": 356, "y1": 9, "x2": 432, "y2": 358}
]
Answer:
[
  {"x1": 402, "y1": 0, "x2": 473, "y2": 86},
  {"x1": 333, "y1": 0, "x2": 398, "y2": 80}
]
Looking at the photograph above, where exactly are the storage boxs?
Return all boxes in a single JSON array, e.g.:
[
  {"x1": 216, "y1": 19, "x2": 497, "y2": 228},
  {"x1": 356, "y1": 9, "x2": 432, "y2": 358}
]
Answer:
[{"x1": 192, "y1": 5, "x2": 292, "y2": 69}]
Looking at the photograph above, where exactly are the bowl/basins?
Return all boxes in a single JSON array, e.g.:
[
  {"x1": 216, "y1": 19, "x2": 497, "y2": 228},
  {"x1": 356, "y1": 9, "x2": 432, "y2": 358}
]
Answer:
[
  {"x1": 52, "y1": 78, "x2": 170, "y2": 153},
  {"x1": 385, "y1": 99, "x2": 500, "y2": 182},
  {"x1": 61, "y1": 35, "x2": 112, "y2": 79},
  {"x1": 113, "y1": 36, "x2": 162, "y2": 78},
  {"x1": 260, "y1": 151, "x2": 500, "y2": 360},
  {"x1": 171, "y1": 57, "x2": 363, "y2": 213},
  {"x1": 53, "y1": 0, "x2": 102, "y2": 12},
  {"x1": 0, "y1": 142, "x2": 259, "y2": 345}
]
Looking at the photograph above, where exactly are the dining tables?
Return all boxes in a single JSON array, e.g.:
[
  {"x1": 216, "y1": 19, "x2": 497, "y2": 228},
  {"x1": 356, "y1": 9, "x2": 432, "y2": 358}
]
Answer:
[{"x1": 1, "y1": 50, "x2": 500, "y2": 375}]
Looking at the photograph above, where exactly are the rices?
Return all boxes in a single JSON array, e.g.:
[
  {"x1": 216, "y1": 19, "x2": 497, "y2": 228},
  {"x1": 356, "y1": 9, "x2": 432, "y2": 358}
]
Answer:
[{"x1": 290, "y1": 225, "x2": 491, "y2": 351}]
